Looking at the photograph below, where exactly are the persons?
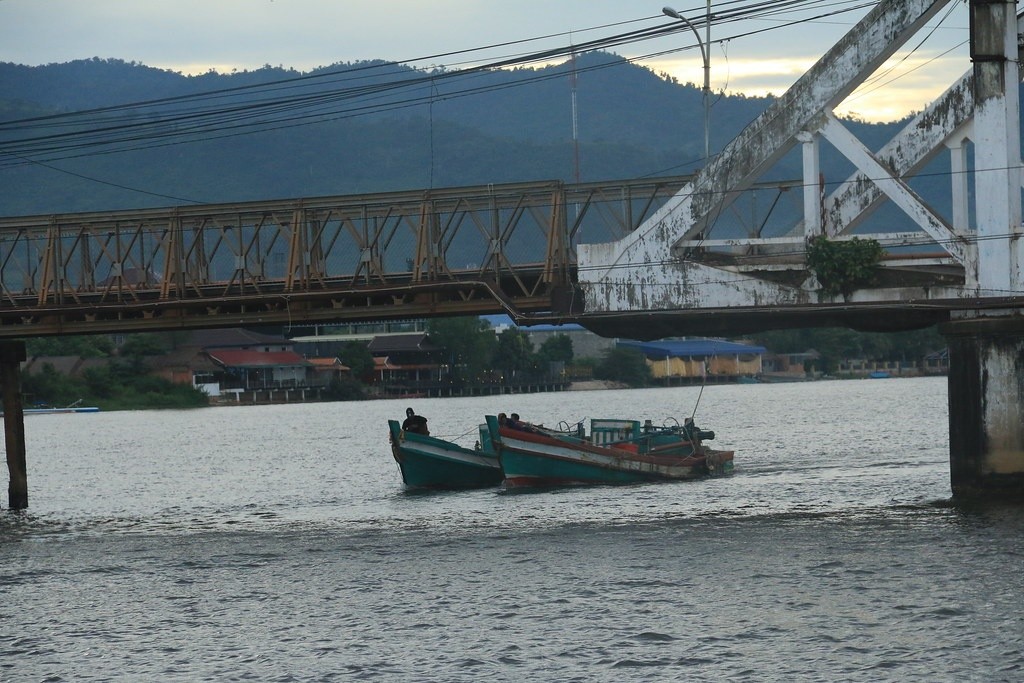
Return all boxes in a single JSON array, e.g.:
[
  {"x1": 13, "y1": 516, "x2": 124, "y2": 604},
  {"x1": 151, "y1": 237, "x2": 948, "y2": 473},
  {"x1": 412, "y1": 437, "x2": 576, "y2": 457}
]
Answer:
[
  {"x1": 498, "y1": 413, "x2": 526, "y2": 431},
  {"x1": 402, "y1": 408, "x2": 429, "y2": 435}
]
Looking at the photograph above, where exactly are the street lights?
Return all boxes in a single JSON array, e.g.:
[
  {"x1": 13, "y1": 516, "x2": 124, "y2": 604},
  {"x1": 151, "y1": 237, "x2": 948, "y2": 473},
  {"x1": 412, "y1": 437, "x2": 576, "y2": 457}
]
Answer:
[{"x1": 661, "y1": 1, "x2": 711, "y2": 181}]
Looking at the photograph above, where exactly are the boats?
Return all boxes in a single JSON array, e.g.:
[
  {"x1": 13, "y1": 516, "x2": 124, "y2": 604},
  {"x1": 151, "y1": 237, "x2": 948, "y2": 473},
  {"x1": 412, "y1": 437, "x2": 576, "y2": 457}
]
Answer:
[
  {"x1": 387, "y1": 420, "x2": 503, "y2": 491},
  {"x1": 0, "y1": 399, "x2": 102, "y2": 417},
  {"x1": 484, "y1": 412, "x2": 735, "y2": 490}
]
